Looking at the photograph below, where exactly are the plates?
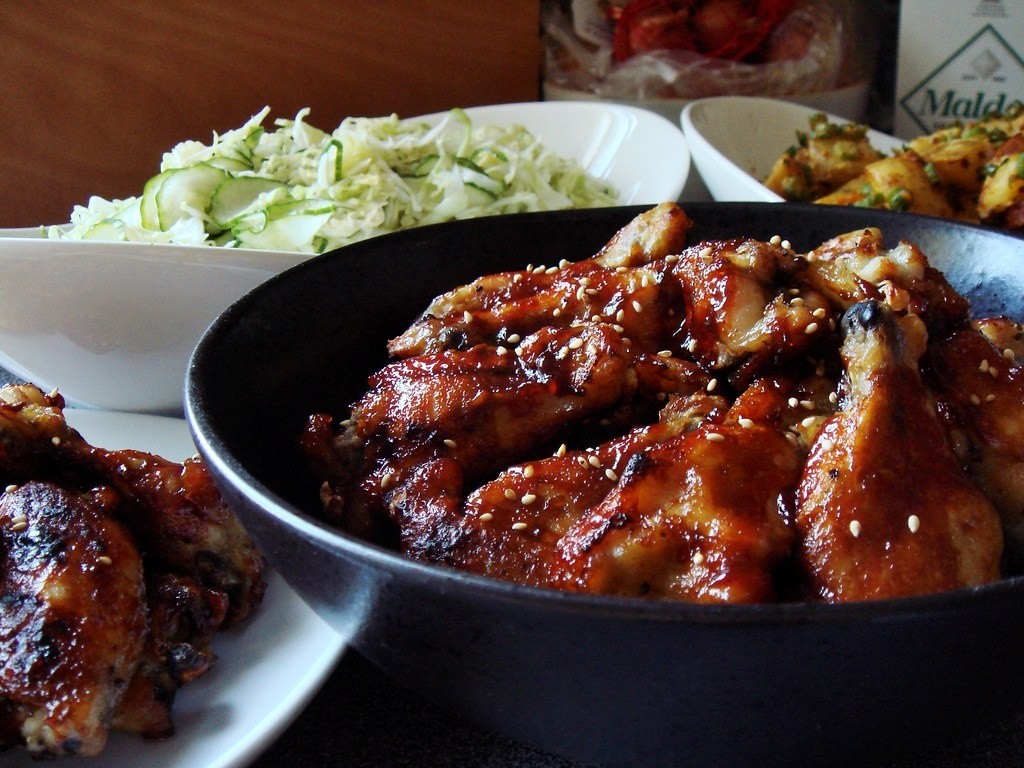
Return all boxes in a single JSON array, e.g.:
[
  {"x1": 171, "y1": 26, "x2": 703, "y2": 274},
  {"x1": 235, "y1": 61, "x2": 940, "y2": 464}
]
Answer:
[{"x1": 0, "y1": 407, "x2": 350, "y2": 768}]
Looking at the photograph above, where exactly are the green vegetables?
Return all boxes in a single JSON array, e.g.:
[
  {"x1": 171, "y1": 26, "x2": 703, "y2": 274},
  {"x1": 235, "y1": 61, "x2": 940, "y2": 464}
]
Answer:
[{"x1": 161, "y1": 103, "x2": 625, "y2": 238}]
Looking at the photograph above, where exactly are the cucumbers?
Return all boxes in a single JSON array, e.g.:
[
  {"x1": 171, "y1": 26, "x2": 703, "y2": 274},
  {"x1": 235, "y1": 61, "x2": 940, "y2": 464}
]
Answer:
[{"x1": 139, "y1": 138, "x2": 355, "y2": 252}]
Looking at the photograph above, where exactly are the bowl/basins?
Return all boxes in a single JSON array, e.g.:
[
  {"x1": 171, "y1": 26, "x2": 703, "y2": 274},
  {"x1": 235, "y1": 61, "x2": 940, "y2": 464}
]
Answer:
[
  {"x1": 185, "y1": 201, "x2": 1024, "y2": 768},
  {"x1": 0, "y1": 97, "x2": 694, "y2": 415},
  {"x1": 679, "y1": 97, "x2": 911, "y2": 202}
]
